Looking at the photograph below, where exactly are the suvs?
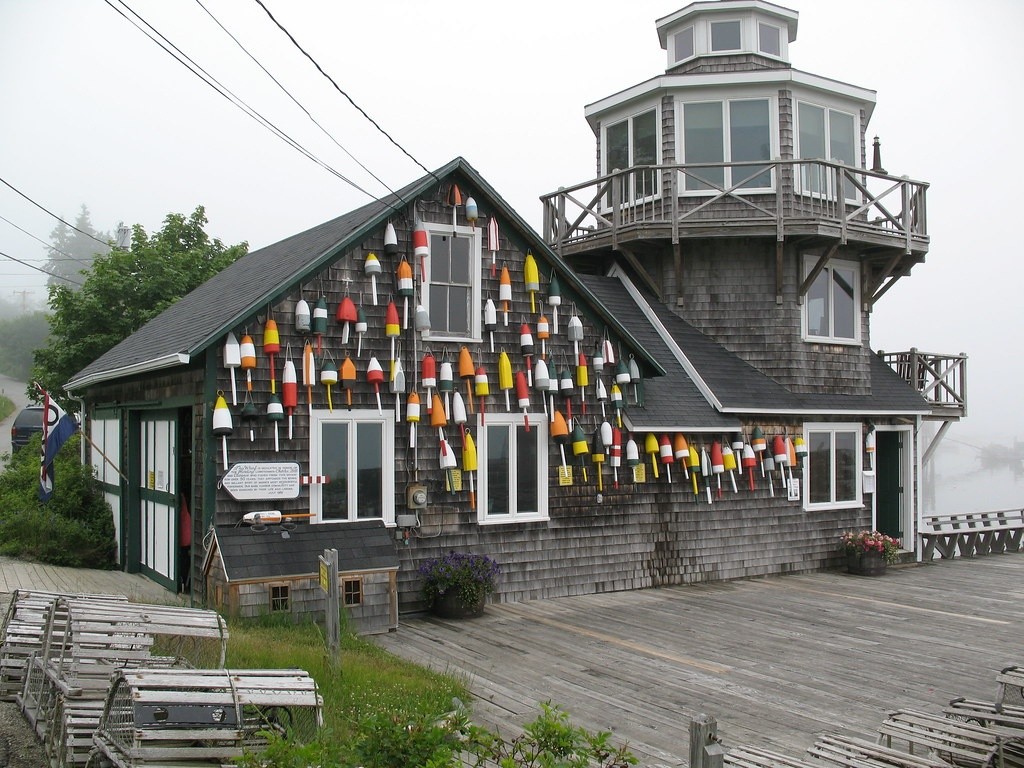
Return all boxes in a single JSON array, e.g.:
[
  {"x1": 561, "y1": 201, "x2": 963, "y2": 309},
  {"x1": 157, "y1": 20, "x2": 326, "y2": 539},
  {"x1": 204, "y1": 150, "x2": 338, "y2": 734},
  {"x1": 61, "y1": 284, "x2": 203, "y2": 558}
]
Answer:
[{"x1": 10, "y1": 403, "x2": 45, "y2": 454}]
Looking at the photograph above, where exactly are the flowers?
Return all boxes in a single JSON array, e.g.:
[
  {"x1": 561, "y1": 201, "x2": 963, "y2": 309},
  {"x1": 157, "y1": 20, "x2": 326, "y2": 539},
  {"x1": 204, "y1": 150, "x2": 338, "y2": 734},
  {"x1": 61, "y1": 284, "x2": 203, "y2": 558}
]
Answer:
[
  {"x1": 835, "y1": 529, "x2": 903, "y2": 566},
  {"x1": 417, "y1": 551, "x2": 502, "y2": 614}
]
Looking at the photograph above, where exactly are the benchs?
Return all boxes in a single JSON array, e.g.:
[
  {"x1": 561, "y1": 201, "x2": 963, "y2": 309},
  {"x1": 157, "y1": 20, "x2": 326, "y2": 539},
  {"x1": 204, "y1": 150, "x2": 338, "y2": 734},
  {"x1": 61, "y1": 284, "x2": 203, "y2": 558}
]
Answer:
[{"x1": 919, "y1": 508, "x2": 1024, "y2": 561}]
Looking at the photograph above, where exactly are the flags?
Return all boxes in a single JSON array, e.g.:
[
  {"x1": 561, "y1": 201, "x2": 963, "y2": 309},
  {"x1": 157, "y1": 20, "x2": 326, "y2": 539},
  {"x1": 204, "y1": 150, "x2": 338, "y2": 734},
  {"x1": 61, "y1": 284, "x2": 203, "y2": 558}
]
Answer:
[{"x1": 38, "y1": 391, "x2": 77, "y2": 504}]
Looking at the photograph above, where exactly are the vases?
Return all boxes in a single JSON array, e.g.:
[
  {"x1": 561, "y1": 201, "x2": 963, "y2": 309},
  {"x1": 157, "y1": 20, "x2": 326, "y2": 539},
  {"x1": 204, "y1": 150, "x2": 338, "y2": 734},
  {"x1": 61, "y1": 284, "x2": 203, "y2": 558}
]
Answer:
[
  {"x1": 433, "y1": 593, "x2": 485, "y2": 619},
  {"x1": 846, "y1": 553, "x2": 888, "y2": 576}
]
